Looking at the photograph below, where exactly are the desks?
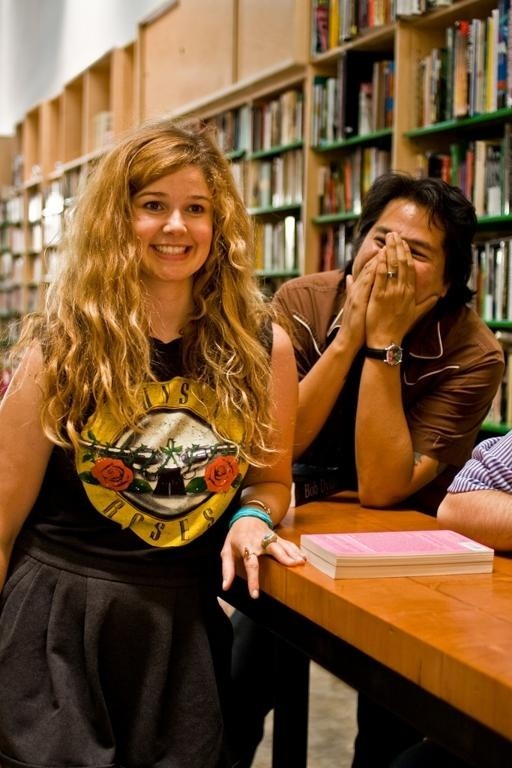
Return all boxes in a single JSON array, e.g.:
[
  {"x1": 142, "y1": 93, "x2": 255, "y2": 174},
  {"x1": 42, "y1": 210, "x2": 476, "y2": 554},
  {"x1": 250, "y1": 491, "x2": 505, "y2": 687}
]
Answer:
[{"x1": 210, "y1": 484, "x2": 512, "y2": 768}]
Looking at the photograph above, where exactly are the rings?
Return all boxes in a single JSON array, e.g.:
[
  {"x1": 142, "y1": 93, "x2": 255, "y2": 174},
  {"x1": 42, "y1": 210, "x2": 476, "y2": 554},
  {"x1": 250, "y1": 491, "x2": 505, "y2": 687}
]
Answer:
[
  {"x1": 261, "y1": 534, "x2": 278, "y2": 549},
  {"x1": 243, "y1": 549, "x2": 258, "y2": 560}
]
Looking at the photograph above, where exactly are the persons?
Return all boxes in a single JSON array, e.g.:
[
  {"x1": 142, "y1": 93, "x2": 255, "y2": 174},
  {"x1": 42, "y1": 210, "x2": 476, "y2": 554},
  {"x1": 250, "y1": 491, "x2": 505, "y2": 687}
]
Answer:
[
  {"x1": 227, "y1": 168, "x2": 507, "y2": 768},
  {"x1": 390, "y1": 427, "x2": 512, "y2": 768},
  {"x1": 2, "y1": 120, "x2": 307, "y2": 768}
]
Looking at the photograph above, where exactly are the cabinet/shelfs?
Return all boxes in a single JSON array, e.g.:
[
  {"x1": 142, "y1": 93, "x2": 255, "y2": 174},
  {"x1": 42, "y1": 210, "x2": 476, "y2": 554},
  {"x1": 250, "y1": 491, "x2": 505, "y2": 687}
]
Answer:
[{"x1": 1, "y1": 0, "x2": 509, "y2": 444}]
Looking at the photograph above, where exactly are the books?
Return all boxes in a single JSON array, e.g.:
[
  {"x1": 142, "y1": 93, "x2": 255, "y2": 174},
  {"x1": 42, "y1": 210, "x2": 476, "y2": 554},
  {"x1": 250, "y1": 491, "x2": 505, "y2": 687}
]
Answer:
[
  {"x1": 1, "y1": 162, "x2": 96, "y2": 383},
  {"x1": 300, "y1": 529, "x2": 495, "y2": 578}
]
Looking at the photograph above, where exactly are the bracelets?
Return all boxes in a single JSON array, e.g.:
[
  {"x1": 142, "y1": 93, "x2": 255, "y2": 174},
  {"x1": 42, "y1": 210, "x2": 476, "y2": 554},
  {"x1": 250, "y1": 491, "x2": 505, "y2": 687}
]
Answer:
[
  {"x1": 244, "y1": 500, "x2": 270, "y2": 515},
  {"x1": 230, "y1": 507, "x2": 273, "y2": 527}
]
